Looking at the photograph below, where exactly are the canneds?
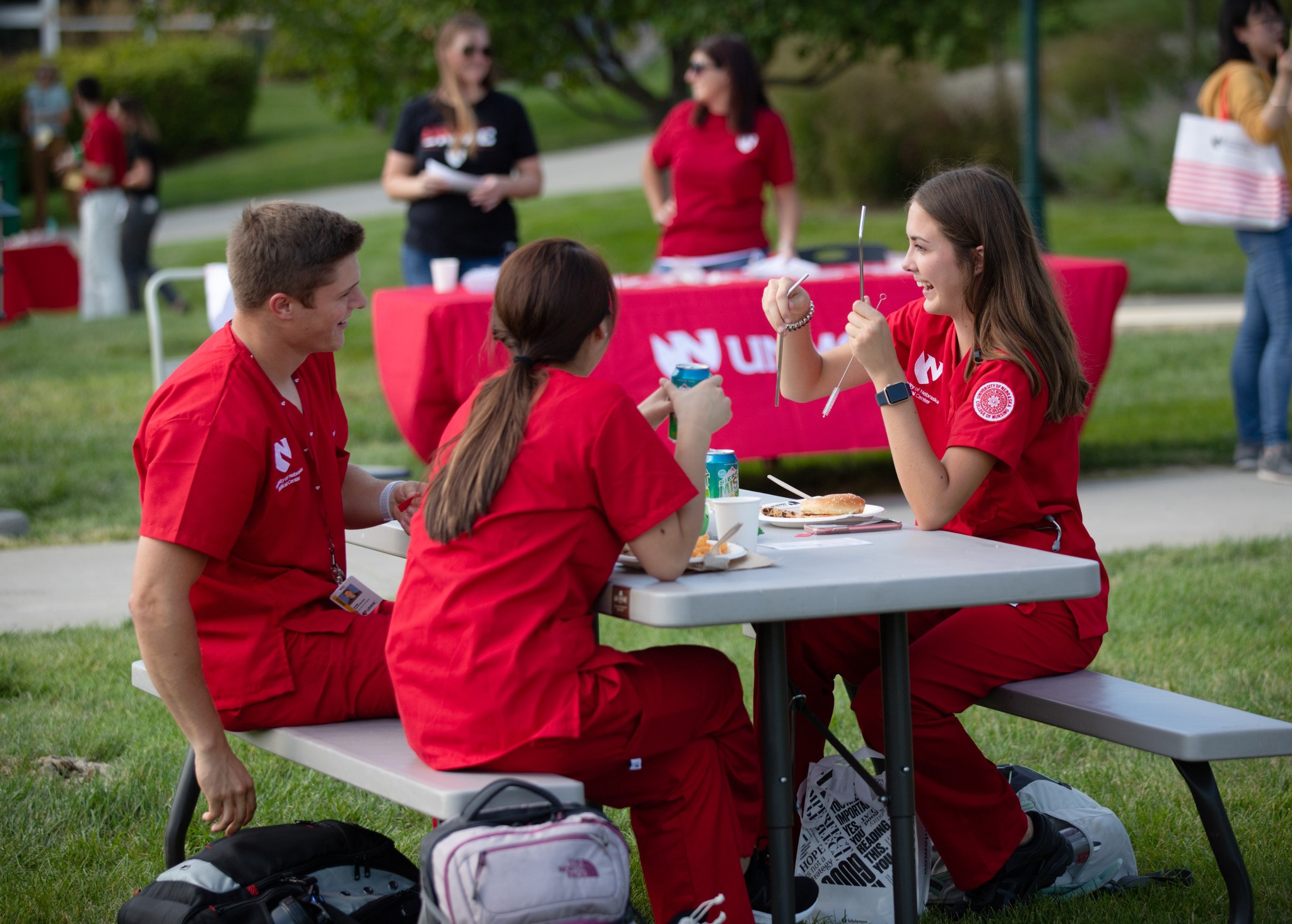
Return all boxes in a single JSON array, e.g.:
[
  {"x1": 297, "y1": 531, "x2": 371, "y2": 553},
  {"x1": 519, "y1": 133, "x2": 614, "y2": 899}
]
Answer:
[
  {"x1": 705, "y1": 448, "x2": 739, "y2": 499},
  {"x1": 668, "y1": 362, "x2": 712, "y2": 443}
]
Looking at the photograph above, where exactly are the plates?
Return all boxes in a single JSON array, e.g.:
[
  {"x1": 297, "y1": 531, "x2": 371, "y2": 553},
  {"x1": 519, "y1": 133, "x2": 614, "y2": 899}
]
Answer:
[
  {"x1": 616, "y1": 539, "x2": 748, "y2": 568},
  {"x1": 759, "y1": 498, "x2": 887, "y2": 529}
]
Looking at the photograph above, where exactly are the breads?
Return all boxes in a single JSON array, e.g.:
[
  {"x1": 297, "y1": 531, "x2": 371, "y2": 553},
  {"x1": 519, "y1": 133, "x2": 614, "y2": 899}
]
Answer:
[{"x1": 800, "y1": 493, "x2": 866, "y2": 518}]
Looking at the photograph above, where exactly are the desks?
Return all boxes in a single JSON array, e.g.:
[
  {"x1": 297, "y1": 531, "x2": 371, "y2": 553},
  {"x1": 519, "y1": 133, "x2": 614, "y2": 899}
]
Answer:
[
  {"x1": 342, "y1": 481, "x2": 1103, "y2": 924},
  {"x1": 366, "y1": 252, "x2": 1132, "y2": 493},
  {"x1": 0, "y1": 229, "x2": 80, "y2": 330}
]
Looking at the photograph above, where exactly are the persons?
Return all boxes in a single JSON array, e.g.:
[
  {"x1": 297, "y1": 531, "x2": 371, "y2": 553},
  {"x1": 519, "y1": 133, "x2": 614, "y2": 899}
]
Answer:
[
  {"x1": 126, "y1": 204, "x2": 430, "y2": 835},
  {"x1": 20, "y1": 56, "x2": 193, "y2": 324},
  {"x1": 754, "y1": 166, "x2": 1109, "y2": 916},
  {"x1": 387, "y1": 239, "x2": 821, "y2": 924},
  {"x1": 644, "y1": 34, "x2": 797, "y2": 278},
  {"x1": 381, "y1": 13, "x2": 541, "y2": 285},
  {"x1": 1195, "y1": 1, "x2": 1292, "y2": 482}
]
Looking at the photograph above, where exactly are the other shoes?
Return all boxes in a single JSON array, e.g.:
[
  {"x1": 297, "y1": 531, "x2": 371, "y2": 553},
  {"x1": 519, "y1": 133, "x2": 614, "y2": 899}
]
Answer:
[{"x1": 1232, "y1": 443, "x2": 1292, "y2": 484}]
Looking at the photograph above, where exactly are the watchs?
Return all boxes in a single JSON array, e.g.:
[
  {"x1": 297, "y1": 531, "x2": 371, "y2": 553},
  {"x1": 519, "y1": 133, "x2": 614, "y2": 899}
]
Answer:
[{"x1": 875, "y1": 382, "x2": 913, "y2": 406}]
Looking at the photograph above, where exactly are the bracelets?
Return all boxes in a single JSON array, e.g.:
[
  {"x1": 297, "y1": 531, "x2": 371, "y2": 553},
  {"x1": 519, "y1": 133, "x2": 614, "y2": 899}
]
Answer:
[
  {"x1": 782, "y1": 300, "x2": 815, "y2": 332},
  {"x1": 379, "y1": 480, "x2": 406, "y2": 521},
  {"x1": 1267, "y1": 97, "x2": 1287, "y2": 108}
]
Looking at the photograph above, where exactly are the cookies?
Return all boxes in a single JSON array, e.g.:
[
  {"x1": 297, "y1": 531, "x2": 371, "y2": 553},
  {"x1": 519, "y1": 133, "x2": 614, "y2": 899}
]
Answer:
[
  {"x1": 621, "y1": 543, "x2": 634, "y2": 556},
  {"x1": 761, "y1": 507, "x2": 804, "y2": 518}
]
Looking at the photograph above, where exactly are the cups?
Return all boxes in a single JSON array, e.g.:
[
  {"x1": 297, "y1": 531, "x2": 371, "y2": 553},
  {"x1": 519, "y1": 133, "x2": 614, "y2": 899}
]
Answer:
[
  {"x1": 712, "y1": 496, "x2": 762, "y2": 553},
  {"x1": 429, "y1": 256, "x2": 460, "y2": 294}
]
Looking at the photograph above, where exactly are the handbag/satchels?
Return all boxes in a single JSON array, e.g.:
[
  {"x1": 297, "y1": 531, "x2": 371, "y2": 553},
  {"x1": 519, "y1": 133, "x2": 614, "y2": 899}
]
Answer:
[
  {"x1": 794, "y1": 747, "x2": 932, "y2": 924},
  {"x1": 1164, "y1": 65, "x2": 1288, "y2": 231}
]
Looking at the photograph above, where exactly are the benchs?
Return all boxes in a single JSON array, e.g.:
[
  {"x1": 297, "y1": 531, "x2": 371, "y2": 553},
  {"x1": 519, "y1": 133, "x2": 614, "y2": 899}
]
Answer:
[
  {"x1": 124, "y1": 648, "x2": 591, "y2": 876},
  {"x1": 976, "y1": 665, "x2": 1292, "y2": 924}
]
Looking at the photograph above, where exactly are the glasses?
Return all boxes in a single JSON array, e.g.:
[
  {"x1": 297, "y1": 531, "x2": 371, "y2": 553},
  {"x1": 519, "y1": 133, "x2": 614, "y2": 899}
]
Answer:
[
  {"x1": 461, "y1": 43, "x2": 494, "y2": 56},
  {"x1": 686, "y1": 62, "x2": 719, "y2": 74}
]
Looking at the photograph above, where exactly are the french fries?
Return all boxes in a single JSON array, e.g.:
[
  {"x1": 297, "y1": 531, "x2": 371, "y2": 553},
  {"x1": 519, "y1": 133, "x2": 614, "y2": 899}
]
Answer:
[{"x1": 692, "y1": 534, "x2": 727, "y2": 558}]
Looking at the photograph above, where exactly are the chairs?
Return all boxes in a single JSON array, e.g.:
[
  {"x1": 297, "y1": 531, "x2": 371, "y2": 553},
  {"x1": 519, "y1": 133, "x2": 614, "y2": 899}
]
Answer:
[{"x1": 141, "y1": 257, "x2": 237, "y2": 399}]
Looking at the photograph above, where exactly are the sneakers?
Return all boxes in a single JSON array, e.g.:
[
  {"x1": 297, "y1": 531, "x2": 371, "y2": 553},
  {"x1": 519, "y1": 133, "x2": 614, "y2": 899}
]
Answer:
[
  {"x1": 952, "y1": 810, "x2": 1073, "y2": 924},
  {"x1": 743, "y1": 855, "x2": 819, "y2": 924}
]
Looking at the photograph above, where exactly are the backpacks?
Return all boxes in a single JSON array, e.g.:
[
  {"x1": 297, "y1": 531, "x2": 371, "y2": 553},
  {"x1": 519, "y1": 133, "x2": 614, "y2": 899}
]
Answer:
[
  {"x1": 416, "y1": 777, "x2": 647, "y2": 924},
  {"x1": 926, "y1": 763, "x2": 1195, "y2": 907},
  {"x1": 118, "y1": 820, "x2": 423, "y2": 924}
]
